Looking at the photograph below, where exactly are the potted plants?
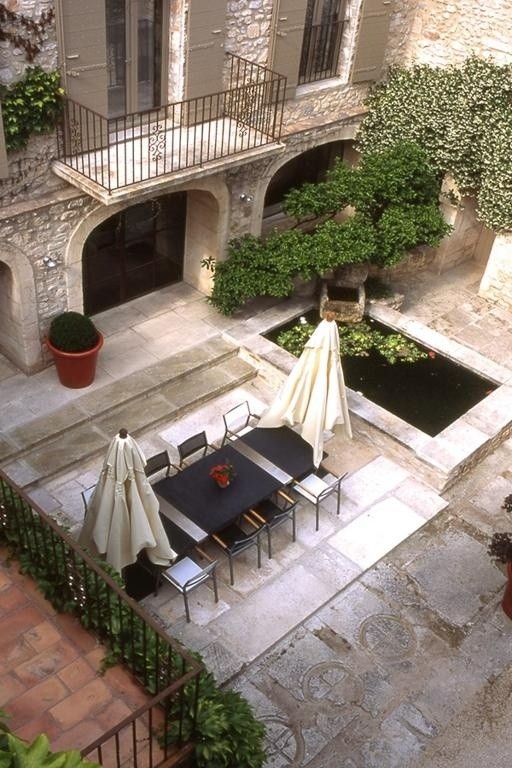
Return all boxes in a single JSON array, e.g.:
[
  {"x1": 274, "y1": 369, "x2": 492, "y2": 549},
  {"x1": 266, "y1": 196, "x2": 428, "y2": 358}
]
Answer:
[
  {"x1": 487, "y1": 494, "x2": 512, "y2": 620},
  {"x1": 46, "y1": 311, "x2": 103, "y2": 389},
  {"x1": 209, "y1": 464, "x2": 237, "y2": 489}
]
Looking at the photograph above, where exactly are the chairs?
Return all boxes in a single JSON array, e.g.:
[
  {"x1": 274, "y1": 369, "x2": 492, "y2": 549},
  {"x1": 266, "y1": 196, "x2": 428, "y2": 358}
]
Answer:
[
  {"x1": 145, "y1": 451, "x2": 183, "y2": 484},
  {"x1": 177, "y1": 430, "x2": 220, "y2": 474},
  {"x1": 154, "y1": 555, "x2": 218, "y2": 622},
  {"x1": 199, "y1": 517, "x2": 266, "y2": 586},
  {"x1": 239, "y1": 486, "x2": 300, "y2": 558},
  {"x1": 282, "y1": 463, "x2": 349, "y2": 531},
  {"x1": 222, "y1": 402, "x2": 261, "y2": 448}
]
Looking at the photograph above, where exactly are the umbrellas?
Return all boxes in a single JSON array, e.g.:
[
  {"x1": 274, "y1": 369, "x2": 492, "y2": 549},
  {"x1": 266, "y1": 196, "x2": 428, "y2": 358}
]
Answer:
[
  {"x1": 258, "y1": 309, "x2": 354, "y2": 468},
  {"x1": 78, "y1": 427, "x2": 181, "y2": 581}
]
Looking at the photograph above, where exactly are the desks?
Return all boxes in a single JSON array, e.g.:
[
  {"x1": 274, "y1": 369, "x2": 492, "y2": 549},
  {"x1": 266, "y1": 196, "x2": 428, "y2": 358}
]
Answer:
[{"x1": 121, "y1": 425, "x2": 329, "y2": 597}]
